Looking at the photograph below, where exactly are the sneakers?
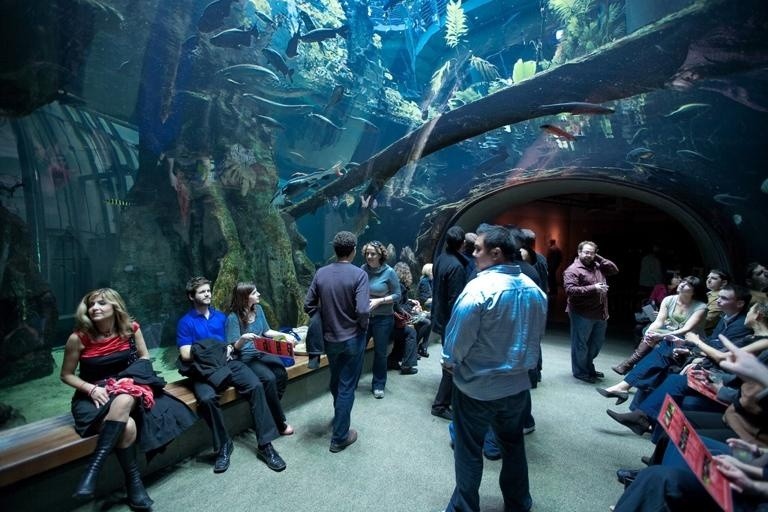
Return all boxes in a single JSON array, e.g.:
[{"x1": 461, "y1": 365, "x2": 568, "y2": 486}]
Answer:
[
  {"x1": 373, "y1": 388, "x2": 384, "y2": 398},
  {"x1": 617, "y1": 468, "x2": 639, "y2": 485}
]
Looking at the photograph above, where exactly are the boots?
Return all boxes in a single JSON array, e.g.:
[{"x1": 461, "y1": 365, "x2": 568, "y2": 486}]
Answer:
[
  {"x1": 69, "y1": 418, "x2": 126, "y2": 502},
  {"x1": 611, "y1": 338, "x2": 654, "y2": 376},
  {"x1": 115, "y1": 433, "x2": 155, "y2": 510}
]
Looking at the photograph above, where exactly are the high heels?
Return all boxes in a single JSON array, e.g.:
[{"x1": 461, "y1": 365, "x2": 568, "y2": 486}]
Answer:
[{"x1": 595, "y1": 386, "x2": 628, "y2": 405}]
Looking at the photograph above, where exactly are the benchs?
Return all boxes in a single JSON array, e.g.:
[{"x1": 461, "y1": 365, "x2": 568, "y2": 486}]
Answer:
[{"x1": 0, "y1": 355, "x2": 315, "y2": 489}]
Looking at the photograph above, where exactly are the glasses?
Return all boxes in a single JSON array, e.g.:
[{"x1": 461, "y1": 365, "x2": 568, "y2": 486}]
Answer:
[{"x1": 581, "y1": 250, "x2": 596, "y2": 256}]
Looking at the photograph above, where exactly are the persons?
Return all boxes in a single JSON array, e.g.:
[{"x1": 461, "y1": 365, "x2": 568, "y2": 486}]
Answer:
[
  {"x1": 564, "y1": 241, "x2": 619, "y2": 384},
  {"x1": 302, "y1": 231, "x2": 433, "y2": 453},
  {"x1": 227, "y1": 281, "x2": 295, "y2": 434},
  {"x1": 744, "y1": 262, "x2": 767, "y2": 306},
  {"x1": 432, "y1": 223, "x2": 550, "y2": 512},
  {"x1": 547, "y1": 239, "x2": 562, "y2": 300},
  {"x1": 596, "y1": 269, "x2": 768, "y2": 512},
  {"x1": 176, "y1": 275, "x2": 286, "y2": 472},
  {"x1": 59, "y1": 289, "x2": 156, "y2": 509}
]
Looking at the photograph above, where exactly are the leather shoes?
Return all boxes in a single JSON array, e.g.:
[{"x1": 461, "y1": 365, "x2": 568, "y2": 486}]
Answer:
[
  {"x1": 213, "y1": 435, "x2": 234, "y2": 474},
  {"x1": 281, "y1": 423, "x2": 294, "y2": 435},
  {"x1": 431, "y1": 406, "x2": 454, "y2": 420},
  {"x1": 386, "y1": 343, "x2": 430, "y2": 375},
  {"x1": 256, "y1": 441, "x2": 286, "y2": 472},
  {"x1": 328, "y1": 428, "x2": 357, "y2": 453},
  {"x1": 642, "y1": 455, "x2": 651, "y2": 463},
  {"x1": 606, "y1": 407, "x2": 650, "y2": 436}
]
